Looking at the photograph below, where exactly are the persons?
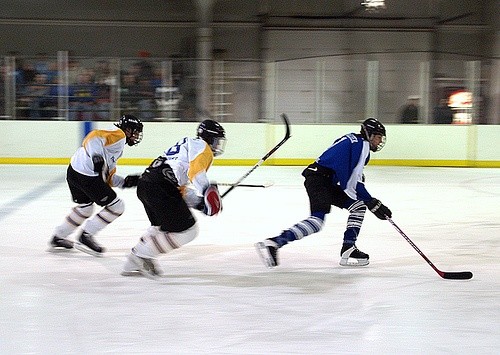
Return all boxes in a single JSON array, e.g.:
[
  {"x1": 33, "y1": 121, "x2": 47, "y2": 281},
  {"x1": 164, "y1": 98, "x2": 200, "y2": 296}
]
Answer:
[
  {"x1": 255, "y1": 118, "x2": 393, "y2": 269},
  {"x1": 48, "y1": 113, "x2": 145, "y2": 258},
  {"x1": 120, "y1": 120, "x2": 228, "y2": 281},
  {"x1": 400, "y1": 93, "x2": 490, "y2": 125},
  {"x1": 0, "y1": 50, "x2": 197, "y2": 123}
]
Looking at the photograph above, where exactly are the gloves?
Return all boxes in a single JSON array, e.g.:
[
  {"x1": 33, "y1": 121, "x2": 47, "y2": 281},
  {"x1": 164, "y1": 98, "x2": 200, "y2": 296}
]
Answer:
[
  {"x1": 193, "y1": 184, "x2": 223, "y2": 216},
  {"x1": 91, "y1": 156, "x2": 104, "y2": 173},
  {"x1": 368, "y1": 199, "x2": 392, "y2": 220},
  {"x1": 122, "y1": 175, "x2": 142, "y2": 188}
]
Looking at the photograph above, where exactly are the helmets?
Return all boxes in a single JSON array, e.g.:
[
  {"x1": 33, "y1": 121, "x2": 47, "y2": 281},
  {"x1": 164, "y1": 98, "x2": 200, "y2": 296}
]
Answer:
[
  {"x1": 360, "y1": 118, "x2": 386, "y2": 139},
  {"x1": 117, "y1": 114, "x2": 143, "y2": 132},
  {"x1": 197, "y1": 120, "x2": 226, "y2": 144}
]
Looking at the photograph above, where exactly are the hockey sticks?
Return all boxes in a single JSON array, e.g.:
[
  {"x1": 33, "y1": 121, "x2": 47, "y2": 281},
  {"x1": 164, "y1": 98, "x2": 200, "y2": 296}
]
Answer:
[
  {"x1": 218, "y1": 180, "x2": 275, "y2": 189},
  {"x1": 220, "y1": 112, "x2": 291, "y2": 198},
  {"x1": 384, "y1": 213, "x2": 473, "y2": 280}
]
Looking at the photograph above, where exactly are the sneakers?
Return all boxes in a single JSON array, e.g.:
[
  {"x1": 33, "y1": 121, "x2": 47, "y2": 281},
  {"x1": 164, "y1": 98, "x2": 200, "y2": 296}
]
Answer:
[
  {"x1": 121, "y1": 252, "x2": 162, "y2": 276},
  {"x1": 339, "y1": 244, "x2": 369, "y2": 266},
  {"x1": 45, "y1": 235, "x2": 75, "y2": 252},
  {"x1": 257, "y1": 238, "x2": 280, "y2": 270},
  {"x1": 73, "y1": 231, "x2": 108, "y2": 258}
]
